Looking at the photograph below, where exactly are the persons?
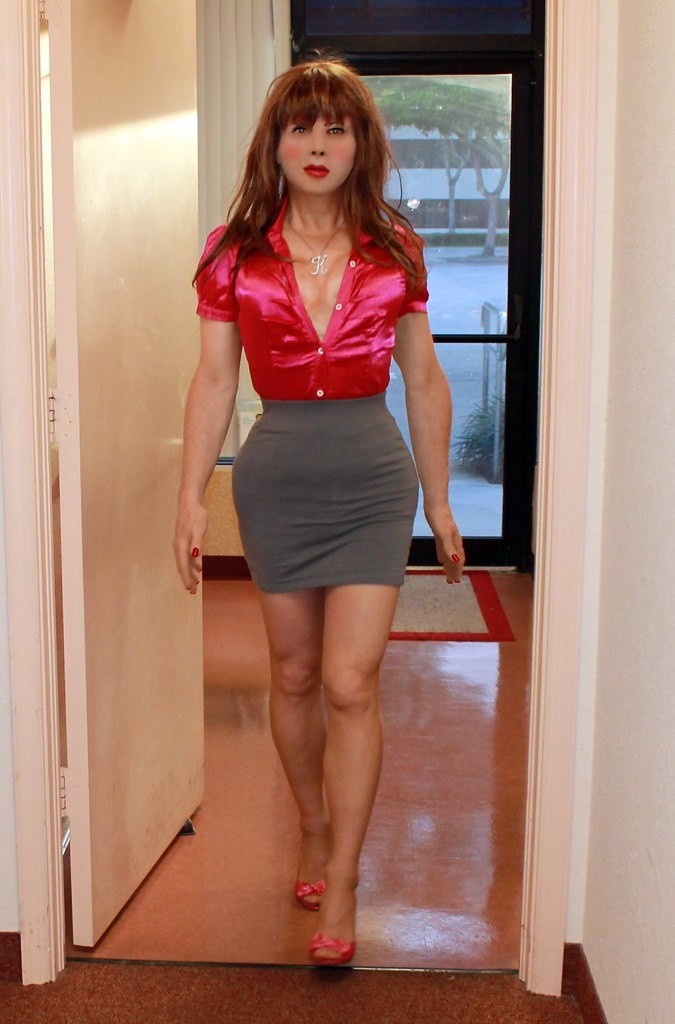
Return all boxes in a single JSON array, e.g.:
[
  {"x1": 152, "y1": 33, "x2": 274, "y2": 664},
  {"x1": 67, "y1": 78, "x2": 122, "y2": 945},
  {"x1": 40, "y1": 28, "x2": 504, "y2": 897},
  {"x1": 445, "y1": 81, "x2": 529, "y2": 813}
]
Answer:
[{"x1": 172, "y1": 60, "x2": 466, "y2": 966}]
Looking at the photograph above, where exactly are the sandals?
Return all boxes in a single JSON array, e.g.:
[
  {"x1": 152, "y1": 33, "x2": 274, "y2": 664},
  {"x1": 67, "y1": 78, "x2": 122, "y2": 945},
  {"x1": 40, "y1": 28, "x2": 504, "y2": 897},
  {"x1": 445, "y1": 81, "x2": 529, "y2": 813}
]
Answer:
[{"x1": 293, "y1": 859, "x2": 357, "y2": 965}]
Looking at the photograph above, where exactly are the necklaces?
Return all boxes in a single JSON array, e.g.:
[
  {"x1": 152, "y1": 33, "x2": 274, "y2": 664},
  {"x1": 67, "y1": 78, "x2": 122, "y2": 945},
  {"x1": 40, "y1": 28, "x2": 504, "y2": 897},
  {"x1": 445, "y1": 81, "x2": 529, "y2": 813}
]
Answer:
[{"x1": 288, "y1": 218, "x2": 347, "y2": 277}]
